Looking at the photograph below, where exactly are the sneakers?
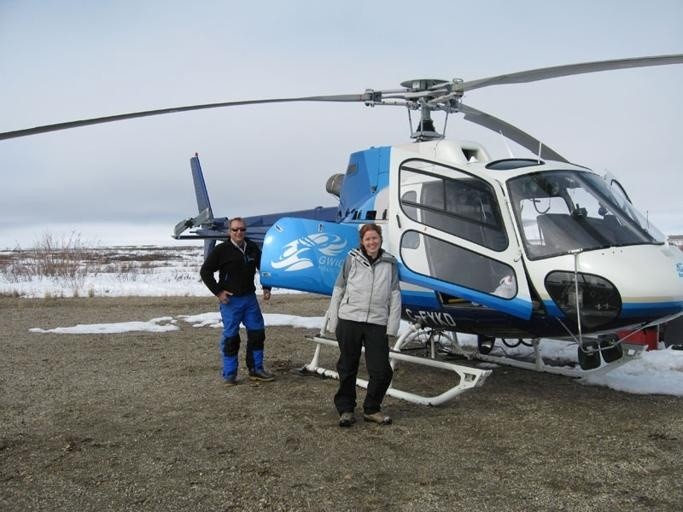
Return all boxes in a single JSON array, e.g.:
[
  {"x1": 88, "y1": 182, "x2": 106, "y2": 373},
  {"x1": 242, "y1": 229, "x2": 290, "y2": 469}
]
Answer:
[
  {"x1": 339, "y1": 412, "x2": 356, "y2": 426},
  {"x1": 364, "y1": 410, "x2": 392, "y2": 425}
]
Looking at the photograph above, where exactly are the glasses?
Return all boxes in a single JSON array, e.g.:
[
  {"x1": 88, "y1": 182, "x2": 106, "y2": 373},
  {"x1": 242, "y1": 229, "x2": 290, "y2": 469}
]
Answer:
[{"x1": 231, "y1": 227, "x2": 247, "y2": 232}]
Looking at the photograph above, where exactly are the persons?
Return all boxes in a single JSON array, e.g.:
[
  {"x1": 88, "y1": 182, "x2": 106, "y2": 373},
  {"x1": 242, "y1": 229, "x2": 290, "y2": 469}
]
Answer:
[
  {"x1": 200, "y1": 217, "x2": 276, "y2": 389},
  {"x1": 328, "y1": 224, "x2": 402, "y2": 426}
]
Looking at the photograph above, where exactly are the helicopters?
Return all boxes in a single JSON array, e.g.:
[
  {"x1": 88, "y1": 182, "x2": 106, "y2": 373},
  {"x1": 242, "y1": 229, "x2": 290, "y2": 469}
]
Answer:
[{"x1": 0, "y1": 53, "x2": 683, "y2": 407}]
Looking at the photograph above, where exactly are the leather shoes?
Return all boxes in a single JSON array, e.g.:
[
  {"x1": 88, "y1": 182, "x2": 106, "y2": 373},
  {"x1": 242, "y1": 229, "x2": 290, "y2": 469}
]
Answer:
[
  {"x1": 249, "y1": 370, "x2": 274, "y2": 381},
  {"x1": 223, "y1": 379, "x2": 236, "y2": 386}
]
